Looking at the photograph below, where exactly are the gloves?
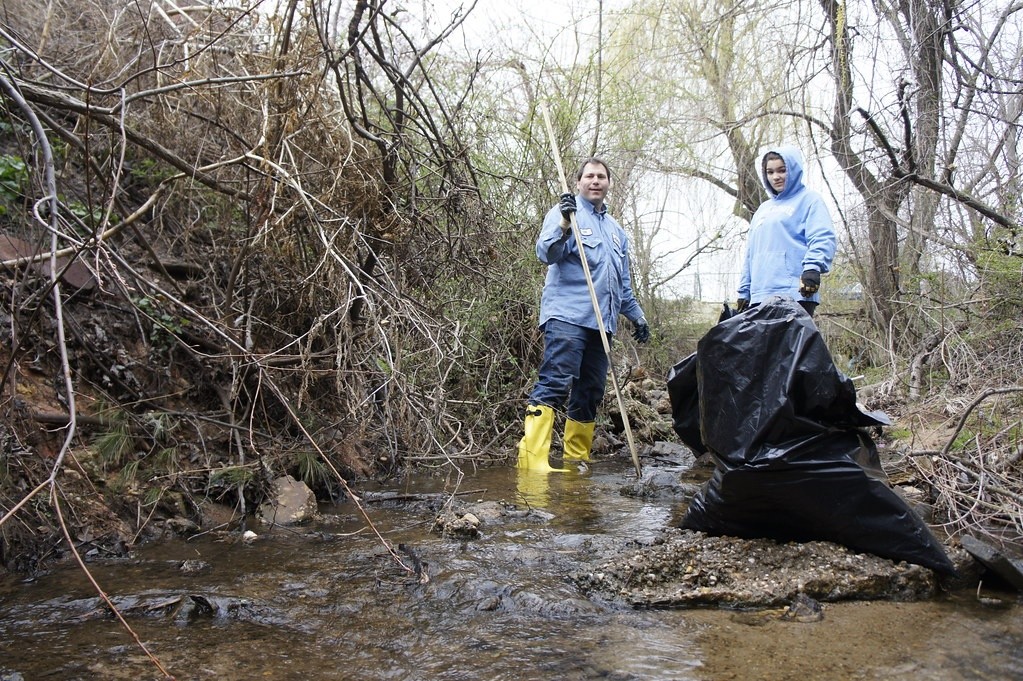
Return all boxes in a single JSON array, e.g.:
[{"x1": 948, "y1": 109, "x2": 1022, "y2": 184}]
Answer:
[
  {"x1": 631, "y1": 316, "x2": 649, "y2": 344},
  {"x1": 559, "y1": 192, "x2": 577, "y2": 222},
  {"x1": 736, "y1": 299, "x2": 749, "y2": 313},
  {"x1": 798, "y1": 269, "x2": 820, "y2": 298}
]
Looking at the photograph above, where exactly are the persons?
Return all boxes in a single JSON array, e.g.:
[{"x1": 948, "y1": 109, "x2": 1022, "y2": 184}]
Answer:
[
  {"x1": 737, "y1": 146, "x2": 836, "y2": 317},
  {"x1": 517, "y1": 158, "x2": 648, "y2": 470}
]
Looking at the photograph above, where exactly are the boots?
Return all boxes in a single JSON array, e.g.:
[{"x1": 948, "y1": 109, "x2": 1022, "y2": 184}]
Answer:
[
  {"x1": 563, "y1": 416, "x2": 596, "y2": 462},
  {"x1": 513, "y1": 405, "x2": 572, "y2": 473}
]
